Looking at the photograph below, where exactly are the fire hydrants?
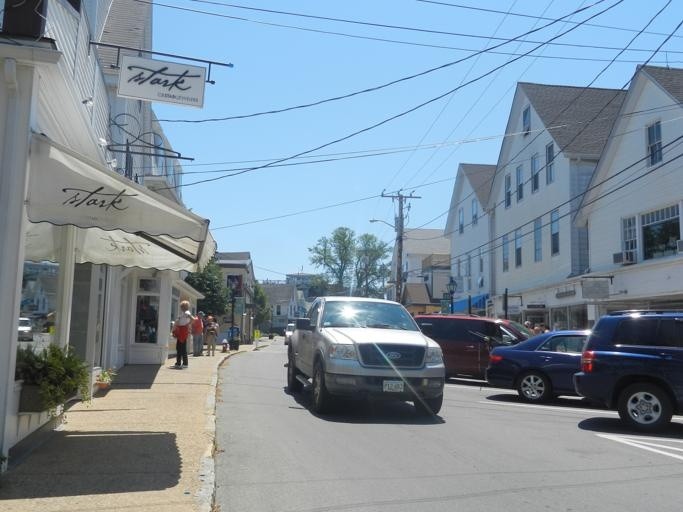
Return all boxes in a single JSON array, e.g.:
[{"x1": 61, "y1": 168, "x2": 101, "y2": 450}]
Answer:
[{"x1": 222, "y1": 339, "x2": 228, "y2": 352}]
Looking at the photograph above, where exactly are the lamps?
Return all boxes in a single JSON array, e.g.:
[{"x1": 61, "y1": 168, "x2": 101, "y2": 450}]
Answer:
[{"x1": 82, "y1": 96, "x2": 118, "y2": 167}]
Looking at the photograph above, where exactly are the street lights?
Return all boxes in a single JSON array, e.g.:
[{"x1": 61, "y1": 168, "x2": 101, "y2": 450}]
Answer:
[
  {"x1": 230, "y1": 283, "x2": 236, "y2": 344},
  {"x1": 249, "y1": 316, "x2": 253, "y2": 341},
  {"x1": 240, "y1": 313, "x2": 247, "y2": 342},
  {"x1": 369, "y1": 219, "x2": 404, "y2": 306},
  {"x1": 270, "y1": 306, "x2": 274, "y2": 335}
]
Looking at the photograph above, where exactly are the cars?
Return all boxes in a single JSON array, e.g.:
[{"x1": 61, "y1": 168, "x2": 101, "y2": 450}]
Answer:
[
  {"x1": 284, "y1": 324, "x2": 296, "y2": 345},
  {"x1": 572, "y1": 307, "x2": 683, "y2": 437},
  {"x1": 484, "y1": 328, "x2": 592, "y2": 405}
]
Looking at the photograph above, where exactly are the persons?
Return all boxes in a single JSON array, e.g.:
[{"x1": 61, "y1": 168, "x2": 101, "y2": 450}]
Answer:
[
  {"x1": 523, "y1": 320, "x2": 550, "y2": 335},
  {"x1": 170, "y1": 300, "x2": 191, "y2": 368},
  {"x1": 191, "y1": 309, "x2": 204, "y2": 357},
  {"x1": 209, "y1": 317, "x2": 218, "y2": 337},
  {"x1": 203, "y1": 315, "x2": 218, "y2": 356}
]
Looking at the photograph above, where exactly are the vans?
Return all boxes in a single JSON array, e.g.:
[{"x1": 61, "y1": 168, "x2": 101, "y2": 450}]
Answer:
[
  {"x1": 17, "y1": 318, "x2": 33, "y2": 340},
  {"x1": 413, "y1": 314, "x2": 533, "y2": 383}
]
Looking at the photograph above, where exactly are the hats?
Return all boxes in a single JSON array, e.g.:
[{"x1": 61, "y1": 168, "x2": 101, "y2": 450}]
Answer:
[{"x1": 199, "y1": 311, "x2": 206, "y2": 317}]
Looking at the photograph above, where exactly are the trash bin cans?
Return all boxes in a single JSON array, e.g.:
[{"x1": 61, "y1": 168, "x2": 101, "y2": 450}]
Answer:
[{"x1": 269, "y1": 334, "x2": 274, "y2": 340}]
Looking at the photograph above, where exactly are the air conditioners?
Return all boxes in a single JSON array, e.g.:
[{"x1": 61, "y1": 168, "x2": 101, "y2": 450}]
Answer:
[
  {"x1": 613, "y1": 250, "x2": 637, "y2": 264},
  {"x1": 676, "y1": 239, "x2": 683, "y2": 253}
]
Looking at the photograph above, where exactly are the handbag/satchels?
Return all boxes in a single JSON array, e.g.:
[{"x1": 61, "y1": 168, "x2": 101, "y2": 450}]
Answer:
[{"x1": 172, "y1": 327, "x2": 180, "y2": 336}]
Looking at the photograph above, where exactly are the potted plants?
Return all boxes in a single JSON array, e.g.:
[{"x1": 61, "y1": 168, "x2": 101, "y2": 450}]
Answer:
[{"x1": 14, "y1": 342, "x2": 117, "y2": 429}]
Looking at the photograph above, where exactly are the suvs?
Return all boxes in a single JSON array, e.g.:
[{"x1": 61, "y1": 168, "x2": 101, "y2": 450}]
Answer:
[{"x1": 286, "y1": 295, "x2": 446, "y2": 416}]
